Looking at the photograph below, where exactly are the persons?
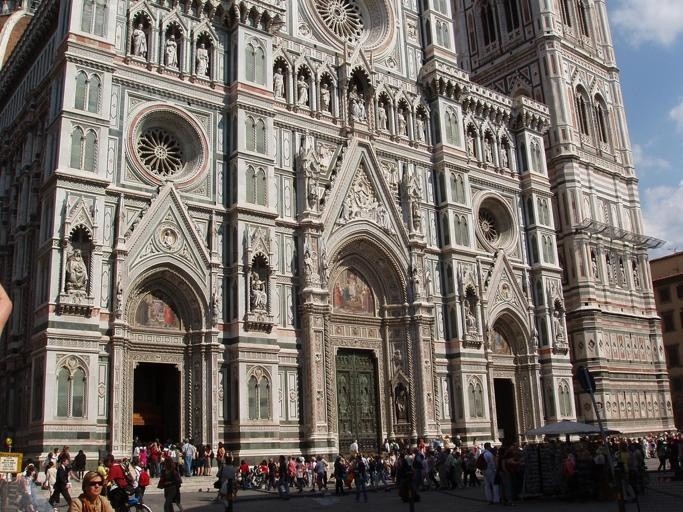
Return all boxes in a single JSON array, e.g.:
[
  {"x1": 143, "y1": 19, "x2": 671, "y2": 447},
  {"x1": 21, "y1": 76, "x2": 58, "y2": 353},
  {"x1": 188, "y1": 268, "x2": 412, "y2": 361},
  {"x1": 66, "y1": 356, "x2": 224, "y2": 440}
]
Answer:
[
  {"x1": 0, "y1": 282, "x2": 13, "y2": 338},
  {"x1": 131, "y1": 23, "x2": 147, "y2": 57},
  {"x1": 500, "y1": 144, "x2": 508, "y2": 167},
  {"x1": 273, "y1": 67, "x2": 285, "y2": 98},
  {"x1": 164, "y1": 33, "x2": 178, "y2": 68},
  {"x1": 66, "y1": 249, "x2": 88, "y2": 293},
  {"x1": 592, "y1": 258, "x2": 640, "y2": 287},
  {"x1": 465, "y1": 299, "x2": 476, "y2": 327},
  {"x1": 297, "y1": 75, "x2": 309, "y2": 105},
  {"x1": 163, "y1": 230, "x2": 174, "y2": 248},
  {"x1": 554, "y1": 311, "x2": 563, "y2": 338},
  {"x1": 349, "y1": 84, "x2": 366, "y2": 122},
  {"x1": 251, "y1": 271, "x2": 267, "y2": 310},
  {"x1": 398, "y1": 109, "x2": 406, "y2": 135},
  {"x1": 1, "y1": 431, "x2": 683, "y2": 512},
  {"x1": 467, "y1": 131, "x2": 474, "y2": 154},
  {"x1": 343, "y1": 181, "x2": 391, "y2": 230},
  {"x1": 195, "y1": 43, "x2": 209, "y2": 77},
  {"x1": 378, "y1": 103, "x2": 387, "y2": 130},
  {"x1": 484, "y1": 139, "x2": 492, "y2": 162},
  {"x1": 417, "y1": 114, "x2": 425, "y2": 142},
  {"x1": 321, "y1": 83, "x2": 330, "y2": 112}
]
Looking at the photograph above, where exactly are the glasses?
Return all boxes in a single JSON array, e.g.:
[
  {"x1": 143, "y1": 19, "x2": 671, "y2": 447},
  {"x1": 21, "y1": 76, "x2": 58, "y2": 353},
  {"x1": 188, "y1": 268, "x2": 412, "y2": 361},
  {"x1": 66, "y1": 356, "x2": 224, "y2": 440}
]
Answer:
[{"x1": 88, "y1": 481, "x2": 103, "y2": 487}]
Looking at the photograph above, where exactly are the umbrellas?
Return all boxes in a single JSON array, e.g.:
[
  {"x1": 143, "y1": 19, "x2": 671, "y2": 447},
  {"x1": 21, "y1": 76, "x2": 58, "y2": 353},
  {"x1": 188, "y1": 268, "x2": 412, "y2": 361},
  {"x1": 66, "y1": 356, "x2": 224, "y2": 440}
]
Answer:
[{"x1": 516, "y1": 419, "x2": 623, "y2": 441}]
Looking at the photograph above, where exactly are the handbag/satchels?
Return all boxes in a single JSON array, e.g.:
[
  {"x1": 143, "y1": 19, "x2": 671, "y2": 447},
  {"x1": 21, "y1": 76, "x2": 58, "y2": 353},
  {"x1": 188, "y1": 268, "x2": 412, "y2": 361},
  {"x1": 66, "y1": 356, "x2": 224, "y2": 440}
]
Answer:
[{"x1": 213, "y1": 479, "x2": 222, "y2": 489}]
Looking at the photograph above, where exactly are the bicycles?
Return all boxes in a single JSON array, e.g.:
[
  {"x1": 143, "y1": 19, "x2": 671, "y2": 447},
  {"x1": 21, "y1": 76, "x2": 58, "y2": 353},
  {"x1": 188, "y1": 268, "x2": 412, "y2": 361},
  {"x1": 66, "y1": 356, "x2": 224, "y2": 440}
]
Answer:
[{"x1": 102, "y1": 485, "x2": 151, "y2": 511}]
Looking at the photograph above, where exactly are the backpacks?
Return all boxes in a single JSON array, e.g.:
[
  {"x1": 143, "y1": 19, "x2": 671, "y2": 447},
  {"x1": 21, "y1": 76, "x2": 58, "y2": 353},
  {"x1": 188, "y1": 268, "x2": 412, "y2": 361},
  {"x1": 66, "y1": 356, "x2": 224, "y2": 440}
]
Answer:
[
  {"x1": 113, "y1": 460, "x2": 136, "y2": 481},
  {"x1": 477, "y1": 452, "x2": 488, "y2": 470}
]
